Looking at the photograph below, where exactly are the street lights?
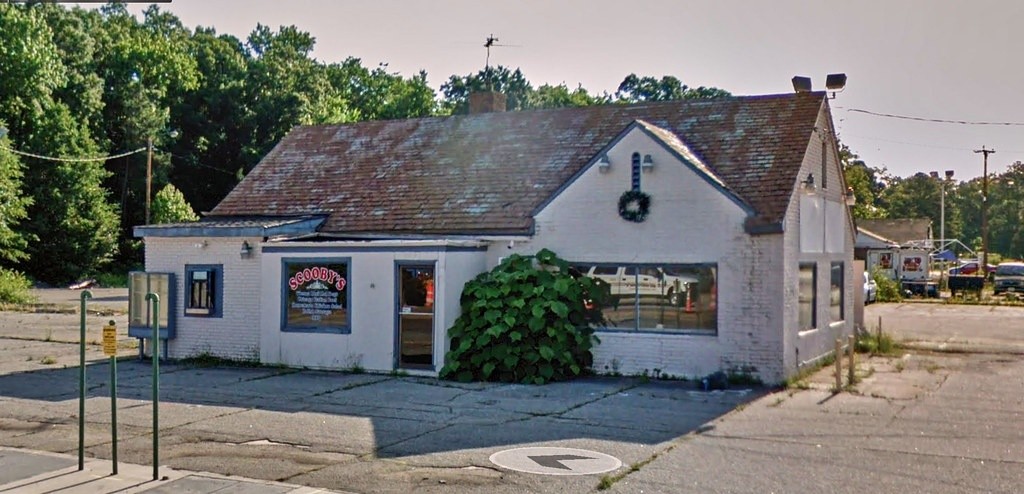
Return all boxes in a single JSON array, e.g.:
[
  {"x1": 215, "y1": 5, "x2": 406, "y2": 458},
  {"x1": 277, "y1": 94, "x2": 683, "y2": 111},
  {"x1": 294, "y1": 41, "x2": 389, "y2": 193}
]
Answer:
[{"x1": 146, "y1": 131, "x2": 179, "y2": 226}]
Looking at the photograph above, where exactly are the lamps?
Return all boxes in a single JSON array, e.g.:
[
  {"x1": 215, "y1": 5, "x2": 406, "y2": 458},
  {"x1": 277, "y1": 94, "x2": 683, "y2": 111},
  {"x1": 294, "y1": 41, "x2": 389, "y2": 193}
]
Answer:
[
  {"x1": 194, "y1": 240, "x2": 207, "y2": 247},
  {"x1": 800, "y1": 174, "x2": 817, "y2": 196},
  {"x1": 240, "y1": 241, "x2": 253, "y2": 259},
  {"x1": 598, "y1": 156, "x2": 609, "y2": 173},
  {"x1": 642, "y1": 155, "x2": 653, "y2": 172},
  {"x1": 825, "y1": 73, "x2": 848, "y2": 99},
  {"x1": 791, "y1": 76, "x2": 813, "y2": 94},
  {"x1": 841, "y1": 187, "x2": 856, "y2": 206}
]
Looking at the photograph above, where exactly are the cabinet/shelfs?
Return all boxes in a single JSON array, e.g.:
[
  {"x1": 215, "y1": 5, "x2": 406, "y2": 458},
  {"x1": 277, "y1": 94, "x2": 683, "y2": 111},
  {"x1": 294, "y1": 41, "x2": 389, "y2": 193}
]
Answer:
[{"x1": 127, "y1": 270, "x2": 176, "y2": 340}]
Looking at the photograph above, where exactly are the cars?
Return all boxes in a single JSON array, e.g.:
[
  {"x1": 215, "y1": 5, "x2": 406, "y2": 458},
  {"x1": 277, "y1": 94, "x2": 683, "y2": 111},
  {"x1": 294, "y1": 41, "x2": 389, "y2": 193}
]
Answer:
[
  {"x1": 862, "y1": 269, "x2": 880, "y2": 306},
  {"x1": 994, "y1": 262, "x2": 1024, "y2": 293},
  {"x1": 948, "y1": 262, "x2": 997, "y2": 274}
]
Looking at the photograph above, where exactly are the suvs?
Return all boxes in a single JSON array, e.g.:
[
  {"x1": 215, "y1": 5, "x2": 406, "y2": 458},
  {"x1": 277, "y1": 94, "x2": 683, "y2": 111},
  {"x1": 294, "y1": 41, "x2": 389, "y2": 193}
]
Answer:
[{"x1": 587, "y1": 263, "x2": 698, "y2": 305}]
